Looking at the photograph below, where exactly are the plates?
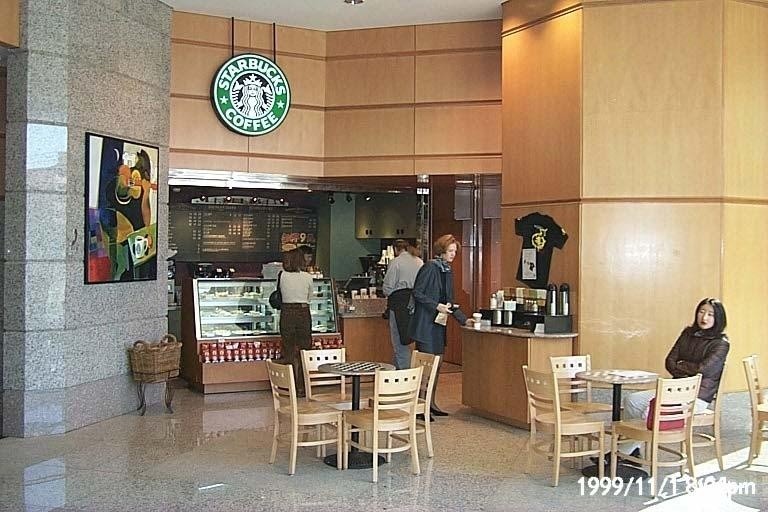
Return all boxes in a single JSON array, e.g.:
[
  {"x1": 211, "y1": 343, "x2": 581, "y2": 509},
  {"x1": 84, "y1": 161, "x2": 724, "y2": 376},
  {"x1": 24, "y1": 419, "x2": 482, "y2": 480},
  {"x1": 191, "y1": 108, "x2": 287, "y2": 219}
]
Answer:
[{"x1": 608, "y1": 371, "x2": 659, "y2": 378}]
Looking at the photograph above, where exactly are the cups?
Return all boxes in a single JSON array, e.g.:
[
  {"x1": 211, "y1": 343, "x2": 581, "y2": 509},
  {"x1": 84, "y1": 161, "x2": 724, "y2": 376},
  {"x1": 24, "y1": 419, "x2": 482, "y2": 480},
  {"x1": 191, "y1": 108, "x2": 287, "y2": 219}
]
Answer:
[
  {"x1": 134, "y1": 236, "x2": 148, "y2": 259},
  {"x1": 473, "y1": 313, "x2": 482, "y2": 329},
  {"x1": 360, "y1": 288, "x2": 367, "y2": 299},
  {"x1": 352, "y1": 290, "x2": 357, "y2": 299},
  {"x1": 369, "y1": 286, "x2": 377, "y2": 298}
]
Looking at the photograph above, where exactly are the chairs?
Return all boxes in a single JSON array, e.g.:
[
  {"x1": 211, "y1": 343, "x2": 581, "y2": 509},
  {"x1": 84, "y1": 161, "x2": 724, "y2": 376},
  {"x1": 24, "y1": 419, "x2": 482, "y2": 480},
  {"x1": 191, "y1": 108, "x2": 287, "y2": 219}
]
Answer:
[
  {"x1": 342, "y1": 365, "x2": 423, "y2": 484},
  {"x1": 522, "y1": 365, "x2": 605, "y2": 487},
  {"x1": 300, "y1": 348, "x2": 365, "y2": 459},
  {"x1": 547, "y1": 353, "x2": 603, "y2": 469},
  {"x1": 265, "y1": 359, "x2": 343, "y2": 476},
  {"x1": 386, "y1": 349, "x2": 440, "y2": 463},
  {"x1": 679, "y1": 358, "x2": 728, "y2": 478},
  {"x1": 742, "y1": 354, "x2": 768, "y2": 469},
  {"x1": 610, "y1": 373, "x2": 702, "y2": 499}
]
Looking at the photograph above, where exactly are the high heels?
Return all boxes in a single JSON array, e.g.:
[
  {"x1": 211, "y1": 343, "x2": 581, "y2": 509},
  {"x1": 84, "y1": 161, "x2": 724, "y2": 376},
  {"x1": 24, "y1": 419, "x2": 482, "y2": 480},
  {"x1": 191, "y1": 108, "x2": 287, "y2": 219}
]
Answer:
[
  {"x1": 430, "y1": 406, "x2": 448, "y2": 416},
  {"x1": 590, "y1": 452, "x2": 619, "y2": 467},
  {"x1": 417, "y1": 414, "x2": 434, "y2": 421},
  {"x1": 624, "y1": 448, "x2": 643, "y2": 466}
]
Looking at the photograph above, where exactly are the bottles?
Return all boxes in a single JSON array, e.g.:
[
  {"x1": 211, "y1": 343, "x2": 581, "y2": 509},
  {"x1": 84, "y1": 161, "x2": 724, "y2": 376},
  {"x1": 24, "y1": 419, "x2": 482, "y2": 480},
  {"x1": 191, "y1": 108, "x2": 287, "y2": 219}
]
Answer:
[
  {"x1": 489, "y1": 293, "x2": 497, "y2": 310},
  {"x1": 546, "y1": 284, "x2": 557, "y2": 315},
  {"x1": 559, "y1": 283, "x2": 570, "y2": 316}
]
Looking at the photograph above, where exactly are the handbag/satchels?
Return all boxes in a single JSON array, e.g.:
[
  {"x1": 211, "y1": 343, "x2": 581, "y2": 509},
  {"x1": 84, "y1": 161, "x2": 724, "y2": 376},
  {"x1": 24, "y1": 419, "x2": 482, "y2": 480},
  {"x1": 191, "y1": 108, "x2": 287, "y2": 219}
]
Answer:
[
  {"x1": 648, "y1": 398, "x2": 685, "y2": 431},
  {"x1": 269, "y1": 270, "x2": 284, "y2": 310},
  {"x1": 389, "y1": 288, "x2": 413, "y2": 313}
]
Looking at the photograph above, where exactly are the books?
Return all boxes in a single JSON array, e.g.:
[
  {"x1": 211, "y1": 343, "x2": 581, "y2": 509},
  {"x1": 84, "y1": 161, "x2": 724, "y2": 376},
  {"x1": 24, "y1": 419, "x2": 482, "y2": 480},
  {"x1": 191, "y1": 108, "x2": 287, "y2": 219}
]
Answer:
[{"x1": 609, "y1": 370, "x2": 660, "y2": 380}]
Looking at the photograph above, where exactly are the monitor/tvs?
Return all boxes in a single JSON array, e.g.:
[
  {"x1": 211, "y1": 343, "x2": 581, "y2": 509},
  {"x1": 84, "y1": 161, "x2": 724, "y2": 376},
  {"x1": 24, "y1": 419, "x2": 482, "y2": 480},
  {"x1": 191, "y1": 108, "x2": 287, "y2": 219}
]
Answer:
[{"x1": 342, "y1": 275, "x2": 372, "y2": 291}]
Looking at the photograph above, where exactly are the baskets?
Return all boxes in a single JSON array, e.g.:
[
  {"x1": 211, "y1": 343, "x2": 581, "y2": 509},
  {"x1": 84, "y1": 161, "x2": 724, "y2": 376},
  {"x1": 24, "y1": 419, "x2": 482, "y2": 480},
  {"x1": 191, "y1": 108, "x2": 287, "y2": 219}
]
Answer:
[{"x1": 128, "y1": 334, "x2": 181, "y2": 380}]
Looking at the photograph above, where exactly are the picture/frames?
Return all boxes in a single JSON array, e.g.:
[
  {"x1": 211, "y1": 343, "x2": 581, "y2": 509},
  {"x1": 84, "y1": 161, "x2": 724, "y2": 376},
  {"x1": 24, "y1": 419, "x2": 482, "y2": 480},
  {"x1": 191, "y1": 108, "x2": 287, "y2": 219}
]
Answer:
[{"x1": 83, "y1": 131, "x2": 159, "y2": 285}]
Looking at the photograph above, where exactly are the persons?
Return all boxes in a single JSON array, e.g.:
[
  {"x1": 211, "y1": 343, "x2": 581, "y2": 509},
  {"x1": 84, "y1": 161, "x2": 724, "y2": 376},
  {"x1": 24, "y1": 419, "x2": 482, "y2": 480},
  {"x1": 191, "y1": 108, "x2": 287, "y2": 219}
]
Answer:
[
  {"x1": 299, "y1": 245, "x2": 313, "y2": 270},
  {"x1": 411, "y1": 233, "x2": 476, "y2": 422},
  {"x1": 382, "y1": 240, "x2": 426, "y2": 370},
  {"x1": 278, "y1": 250, "x2": 313, "y2": 399},
  {"x1": 590, "y1": 298, "x2": 730, "y2": 467}
]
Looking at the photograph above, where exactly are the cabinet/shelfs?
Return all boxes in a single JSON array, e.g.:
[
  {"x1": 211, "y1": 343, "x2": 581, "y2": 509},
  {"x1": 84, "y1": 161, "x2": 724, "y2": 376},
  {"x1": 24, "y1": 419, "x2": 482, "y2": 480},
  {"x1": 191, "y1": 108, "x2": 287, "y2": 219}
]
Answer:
[
  {"x1": 183, "y1": 275, "x2": 343, "y2": 362},
  {"x1": 340, "y1": 296, "x2": 395, "y2": 364},
  {"x1": 460, "y1": 323, "x2": 580, "y2": 431}
]
